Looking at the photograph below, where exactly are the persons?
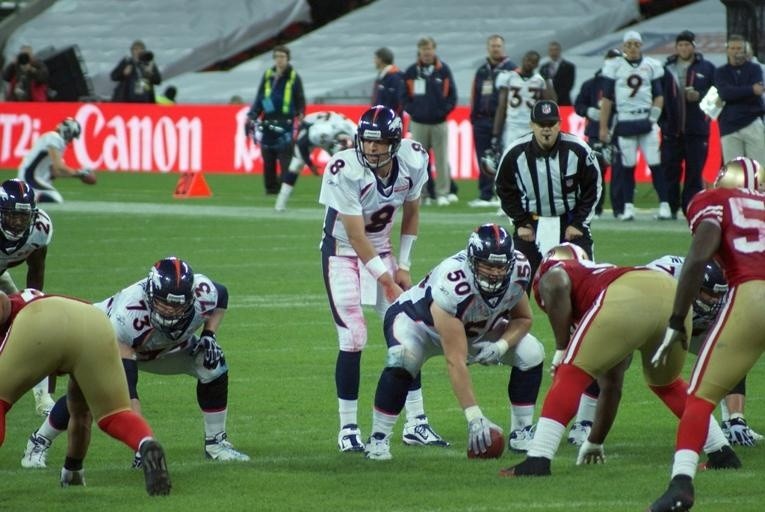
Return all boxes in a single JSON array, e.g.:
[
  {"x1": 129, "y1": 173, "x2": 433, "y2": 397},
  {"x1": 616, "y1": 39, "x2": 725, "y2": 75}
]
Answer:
[
  {"x1": 157, "y1": 86, "x2": 176, "y2": 104},
  {"x1": 1, "y1": 178, "x2": 54, "y2": 295},
  {"x1": 22, "y1": 258, "x2": 251, "y2": 468},
  {"x1": 472, "y1": 37, "x2": 519, "y2": 207},
  {"x1": 373, "y1": 48, "x2": 407, "y2": 117},
  {"x1": 566, "y1": 254, "x2": 762, "y2": 449},
  {"x1": 110, "y1": 40, "x2": 161, "y2": 102},
  {"x1": 541, "y1": 42, "x2": 574, "y2": 105},
  {"x1": 3, "y1": 48, "x2": 51, "y2": 103},
  {"x1": 243, "y1": 46, "x2": 305, "y2": 196},
  {"x1": 599, "y1": 32, "x2": 666, "y2": 222},
  {"x1": 574, "y1": 50, "x2": 620, "y2": 214},
  {"x1": 660, "y1": 33, "x2": 716, "y2": 218},
  {"x1": 406, "y1": 39, "x2": 457, "y2": 206},
  {"x1": 496, "y1": 100, "x2": 603, "y2": 298},
  {"x1": 501, "y1": 244, "x2": 743, "y2": 477},
  {"x1": 273, "y1": 110, "x2": 357, "y2": 209},
  {"x1": 716, "y1": 34, "x2": 764, "y2": 167},
  {"x1": 365, "y1": 228, "x2": 545, "y2": 461},
  {"x1": 650, "y1": 157, "x2": 765, "y2": 512},
  {"x1": 490, "y1": 50, "x2": 548, "y2": 155},
  {"x1": 320, "y1": 104, "x2": 450, "y2": 453},
  {"x1": 3, "y1": 287, "x2": 169, "y2": 496},
  {"x1": 18, "y1": 117, "x2": 95, "y2": 206}
]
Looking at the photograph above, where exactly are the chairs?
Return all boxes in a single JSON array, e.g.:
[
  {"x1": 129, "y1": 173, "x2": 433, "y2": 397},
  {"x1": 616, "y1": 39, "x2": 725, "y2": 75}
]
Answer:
[
  {"x1": 469, "y1": 197, "x2": 503, "y2": 207},
  {"x1": 204, "y1": 432, "x2": 251, "y2": 463},
  {"x1": 140, "y1": 440, "x2": 171, "y2": 497},
  {"x1": 337, "y1": 425, "x2": 364, "y2": 452},
  {"x1": 365, "y1": 432, "x2": 391, "y2": 460},
  {"x1": 35, "y1": 396, "x2": 56, "y2": 416},
  {"x1": 695, "y1": 448, "x2": 742, "y2": 471},
  {"x1": 21, "y1": 432, "x2": 48, "y2": 468},
  {"x1": 657, "y1": 201, "x2": 670, "y2": 218},
  {"x1": 509, "y1": 425, "x2": 537, "y2": 452},
  {"x1": 275, "y1": 200, "x2": 285, "y2": 213},
  {"x1": 624, "y1": 204, "x2": 634, "y2": 220},
  {"x1": 437, "y1": 196, "x2": 448, "y2": 205},
  {"x1": 499, "y1": 455, "x2": 551, "y2": 477},
  {"x1": 650, "y1": 473, "x2": 694, "y2": 512},
  {"x1": 570, "y1": 423, "x2": 596, "y2": 445},
  {"x1": 403, "y1": 417, "x2": 449, "y2": 447},
  {"x1": 447, "y1": 193, "x2": 457, "y2": 202},
  {"x1": 617, "y1": 214, "x2": 624, "y2": 221}
]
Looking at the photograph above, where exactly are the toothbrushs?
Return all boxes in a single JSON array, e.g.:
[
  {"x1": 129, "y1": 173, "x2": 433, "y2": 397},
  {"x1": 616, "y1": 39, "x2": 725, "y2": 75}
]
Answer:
[{"x1": 467, "y1": 427, "x2": 504, "y2": 459}]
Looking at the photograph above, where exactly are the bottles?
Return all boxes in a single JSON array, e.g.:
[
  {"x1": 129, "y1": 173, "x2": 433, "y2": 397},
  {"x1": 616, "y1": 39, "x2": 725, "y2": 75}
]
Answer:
[
  {"x1": 624, "y1": 31, "x2": 642, "y2": 42},
  {"x1": 531, "y1": 101, "x2": 560, "y2": 123},
  {"x1": 676, "y1": 30, "x2": 695, "y2": 45}
]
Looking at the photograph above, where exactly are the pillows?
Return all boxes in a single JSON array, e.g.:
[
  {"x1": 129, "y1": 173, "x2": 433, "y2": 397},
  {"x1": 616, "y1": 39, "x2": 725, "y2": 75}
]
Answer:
[
  {"x1": 307, "y1": 121, "x2": 332, "y2": 149},
  {"x1": 469, "y1": 225, "x2": 514, "y2": 297},
  {"x1": 543, "y1": 243, "x2": 588, "y2": 262},
  {"x1": 20, "y1": 288, "x2": 46, "y2": 299},
  {"x1": 714, "y1": 157, "x2": 764, "y2": 192},
  {"x1": 56, "y1": 117, "x2": 81, "y2": 141},
  {"x1": 146, "y1": 257, "x2": 194, "y2": 330},
  {"x1": 354, "y1": 105, "x2": 402, "y2": 167},
  {"x1": 692, "y1": 264, "x2": 728, "y2": 320},
  {"x1": 0, "y1": 179, "x2": 37, "y2": 244}
]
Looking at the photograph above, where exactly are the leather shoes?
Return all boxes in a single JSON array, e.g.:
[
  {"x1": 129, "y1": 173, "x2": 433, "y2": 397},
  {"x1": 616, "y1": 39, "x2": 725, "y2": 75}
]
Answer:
[
  {"x1": 190, "y1": 331, "x2": 225, "y2": 369},
  {"x1": 491, "y1": 134, "x2": 500, "y2": 151},
  {"x1": 465, "y1": 340, "x2": 508, "y2": 367},
  {"x1": 59, "y1": 458, "x2": 85, "y2": 488},
  {"x1": 651, "y1": 325, "x2": 688, "y2": 369},
  {"x1": 244, "y1": 118, "x2": 255, "y2": 135},
  {"x1": 729, "y1": 419, "x2": 755, "y2": 447},
  {"x1": 576, "y1": 439, "x2": 605, "y2": 466},
  {"x1": 465, "y1": 407, "x2": 504, "y2": 456},
  {"x1": 549, "y1": 349, "x2": 570, "y2": 380},
  {"x1": 74, "y1": 169, "x2": 89, "y2": 176},
  {"x1": 133, "y1": 451, "x2": 144, "y2": 468}
]
